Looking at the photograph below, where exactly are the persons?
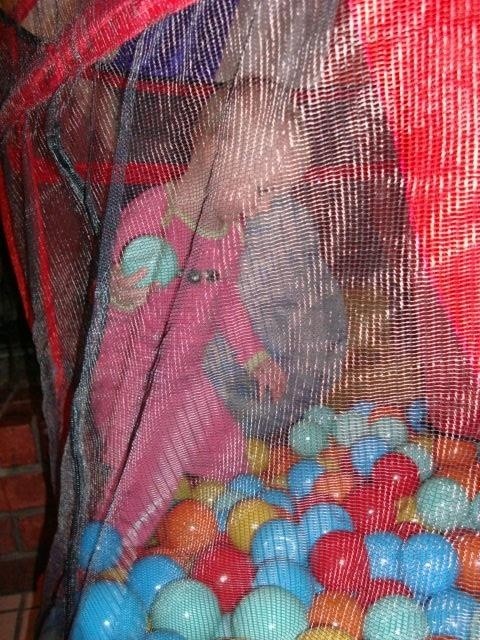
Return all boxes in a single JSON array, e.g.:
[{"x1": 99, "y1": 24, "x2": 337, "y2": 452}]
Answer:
[{"x1": 78, "y1": 75, "x2": 312, "y2": 588}]
